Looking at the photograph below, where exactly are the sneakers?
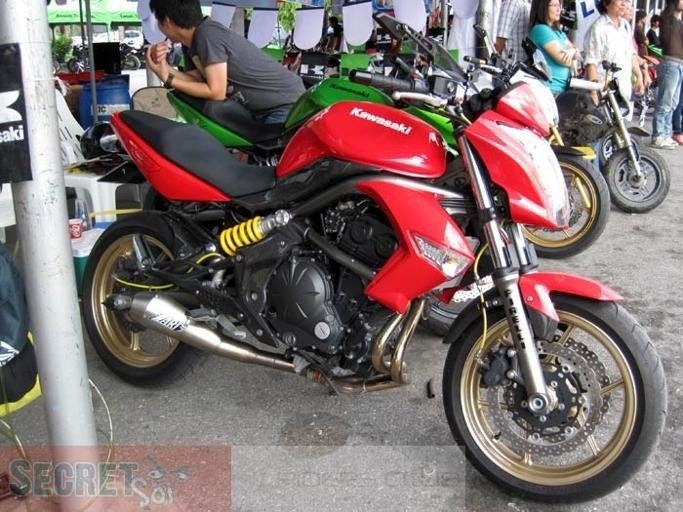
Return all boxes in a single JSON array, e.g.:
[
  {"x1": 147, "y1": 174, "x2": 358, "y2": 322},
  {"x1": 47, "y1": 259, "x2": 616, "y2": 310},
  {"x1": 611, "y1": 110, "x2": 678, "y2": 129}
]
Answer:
[{"x1": 651, "y1": 134, "x2": 683, "y2": 149}]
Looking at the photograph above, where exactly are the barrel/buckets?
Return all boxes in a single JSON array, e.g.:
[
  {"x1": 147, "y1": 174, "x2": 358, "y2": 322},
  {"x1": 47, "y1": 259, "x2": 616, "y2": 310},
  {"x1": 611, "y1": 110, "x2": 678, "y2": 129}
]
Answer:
[{"x1": 79, "y1": 79, "x2": 132, "y2": 130}]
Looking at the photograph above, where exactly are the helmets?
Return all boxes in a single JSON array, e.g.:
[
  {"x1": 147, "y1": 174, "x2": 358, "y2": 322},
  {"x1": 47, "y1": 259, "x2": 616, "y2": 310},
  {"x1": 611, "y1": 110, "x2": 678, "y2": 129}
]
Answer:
[{"x1": 80, "y1": 121, "x2": 129, "y2": 160}]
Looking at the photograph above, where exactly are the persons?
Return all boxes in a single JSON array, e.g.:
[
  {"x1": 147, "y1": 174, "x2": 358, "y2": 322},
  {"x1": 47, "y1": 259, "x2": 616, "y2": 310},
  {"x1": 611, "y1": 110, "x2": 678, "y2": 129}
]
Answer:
[
  {"x1": 582, "y1": 0, "x2": 683, "y2": 150},
  {"x1": 491, "y1": 0, "x2": 532, "y2": 89},
  {"x1": 328, "y1": 16, "x2": 344, "y2": 37},
  {"x1": 145, "y1": 0, "x2": 306, "y2": 124},
  {"x1": 527, "y1": 0, "x2": 581, "y2": 99}
]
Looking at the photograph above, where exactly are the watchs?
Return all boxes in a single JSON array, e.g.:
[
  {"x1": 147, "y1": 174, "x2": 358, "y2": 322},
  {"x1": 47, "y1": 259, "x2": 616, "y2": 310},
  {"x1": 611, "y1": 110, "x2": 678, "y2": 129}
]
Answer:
[{"x1": 163, "y1": 72, "x2": 175, "y2": 89}]
